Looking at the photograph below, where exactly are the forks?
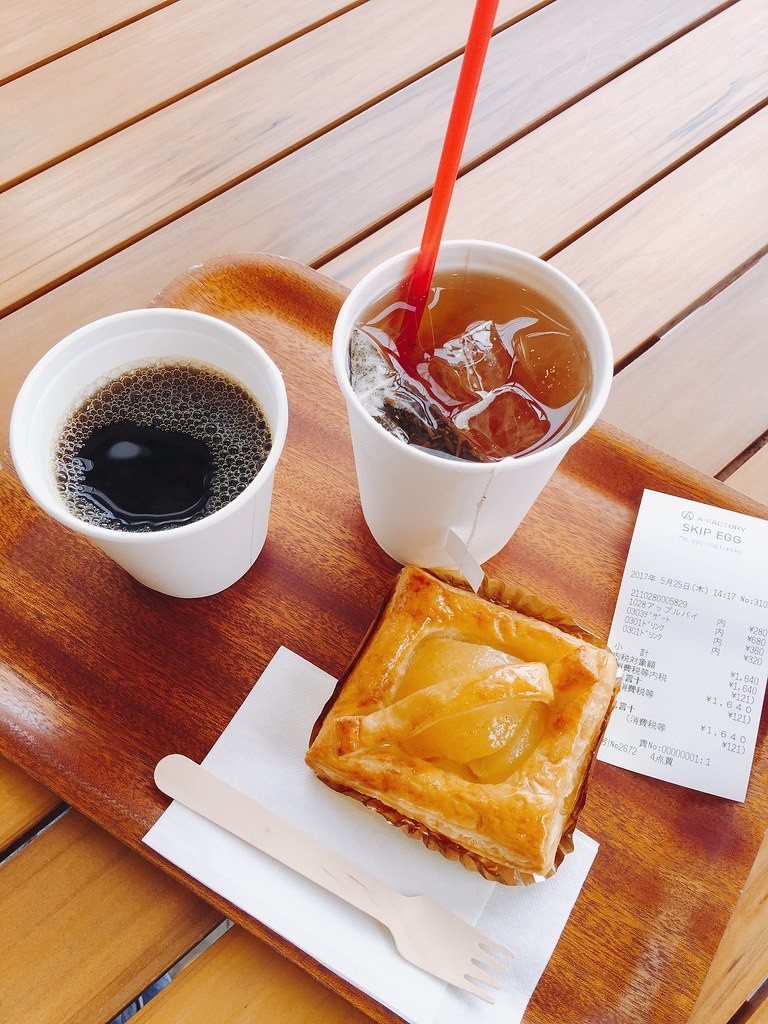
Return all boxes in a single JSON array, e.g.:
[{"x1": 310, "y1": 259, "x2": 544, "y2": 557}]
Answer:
[{"x1": 151, "y1": 751, "x2": 519, "y2": 1006}]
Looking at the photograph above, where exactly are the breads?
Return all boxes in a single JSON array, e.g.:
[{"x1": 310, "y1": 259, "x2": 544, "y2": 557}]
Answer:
[{"x1": 306, "y1": 568, "x2": 617, "y2": 875}]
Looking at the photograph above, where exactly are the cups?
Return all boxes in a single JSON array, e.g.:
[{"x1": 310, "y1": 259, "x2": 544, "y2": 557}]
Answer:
[
  {"x1": 330, "y1": 237, "x2": 615, "y2": 571},
  {"x1": 7, "y1": 306, "x2": 291, "y2": 600}
]
